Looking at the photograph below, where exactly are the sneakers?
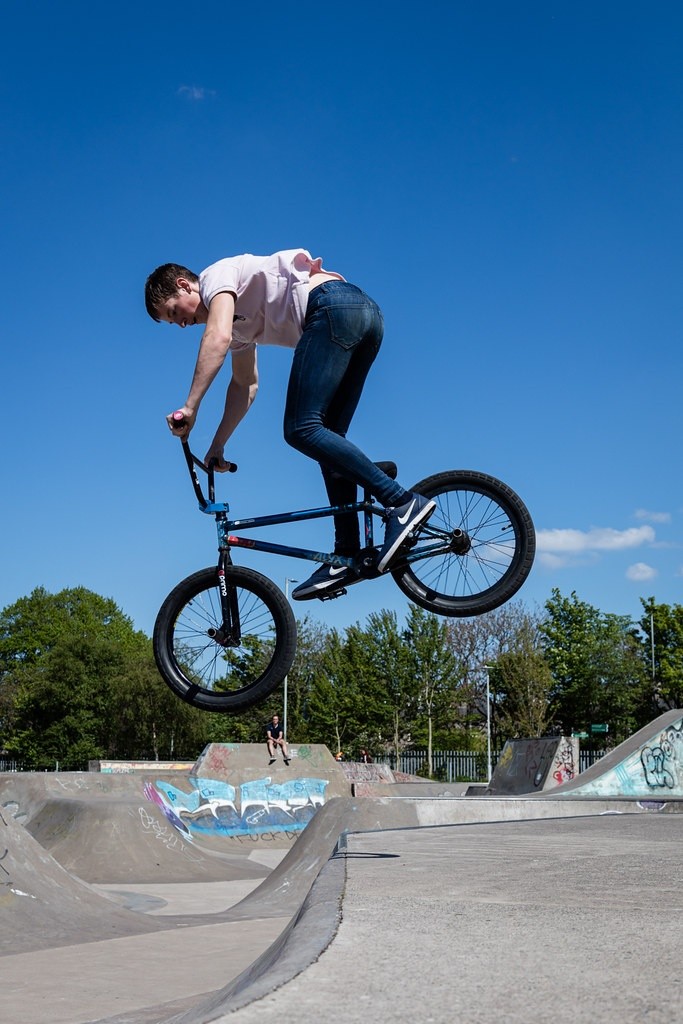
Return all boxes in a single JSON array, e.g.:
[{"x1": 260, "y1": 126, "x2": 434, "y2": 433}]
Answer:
[
  {"x1": 377, "y1": 493, "x2": 436, "y2": 573},
  {"x1": 292, "y1": 563, "x2": 364, "y2": 601}
]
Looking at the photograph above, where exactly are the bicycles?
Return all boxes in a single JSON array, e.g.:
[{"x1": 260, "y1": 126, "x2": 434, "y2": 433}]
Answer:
[{"x1": 149, "y1": 410, "x2": 528, "y2": 717}]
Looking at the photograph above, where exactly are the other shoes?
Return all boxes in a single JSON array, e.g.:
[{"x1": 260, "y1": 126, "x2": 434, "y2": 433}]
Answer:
[
  {"x1": 283, "y1": 756, "x2": 292, "y2": 761},
  {"x1": 270, "y1": 757, "x2": 276, "y2": 760}
]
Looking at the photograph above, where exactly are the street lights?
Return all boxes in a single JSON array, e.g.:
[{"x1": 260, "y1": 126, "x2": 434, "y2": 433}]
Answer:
[
  {"x1": 480, "y1": 663, "x2": 496, "y2": 784},
  {"x1": 279, "y1": 578, "x2": 302, "y2": 744}
]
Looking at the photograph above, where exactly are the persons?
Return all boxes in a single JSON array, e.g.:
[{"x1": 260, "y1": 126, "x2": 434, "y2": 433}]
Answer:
[
  {"x1": 266, "y1": 715, "x2": 291, "y2": 761},
  {"x1": 145, "y1": 247, "x2": 437, "y2": 601}
]
